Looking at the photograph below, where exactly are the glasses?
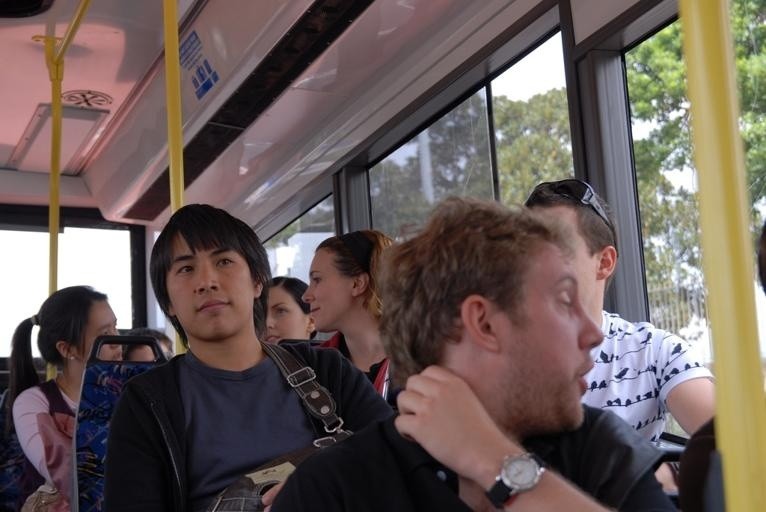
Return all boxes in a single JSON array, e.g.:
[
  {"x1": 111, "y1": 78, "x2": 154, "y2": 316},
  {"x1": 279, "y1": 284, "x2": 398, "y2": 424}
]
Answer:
[{"x1": 534, "y1": 178, "x2": 619, "y2": 258}]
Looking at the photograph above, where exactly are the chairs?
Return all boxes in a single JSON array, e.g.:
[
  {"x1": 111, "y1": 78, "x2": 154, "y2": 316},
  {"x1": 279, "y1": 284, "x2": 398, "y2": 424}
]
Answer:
[{"x1": 74, "y1": 334, "x2": 167, "y2": 512}]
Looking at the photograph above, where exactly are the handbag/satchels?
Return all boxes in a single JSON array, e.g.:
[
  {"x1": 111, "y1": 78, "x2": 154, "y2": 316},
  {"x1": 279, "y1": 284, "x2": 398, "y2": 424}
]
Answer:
[
  {"x1": 204, "y1": 428, "x2": 356, "y2": 512},
  {"x1": 18, "y1": 481, "x2": 64, "y2": 512}
]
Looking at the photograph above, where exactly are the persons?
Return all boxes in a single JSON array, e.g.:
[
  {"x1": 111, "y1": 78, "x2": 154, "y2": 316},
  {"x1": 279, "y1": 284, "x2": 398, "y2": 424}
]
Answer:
[
  {"x1": 266, "y1": 275, "x2": 316, "y2": 344},
  {"x1": 120, "y1": 324, "x2": 176, "y2": 362},
  {"x1": 101, "y1": 202, "x2": 395, "y2": 511},
  {"x1": 269, "y1": 193, "x2": 681, "y2": 512},
  {"x1": 523, "y1": 181, "x2": 718, "y2": 495},
  {"x1": 1, "y1": 284, "x2": 124, "y2": 512},
  {"x1": 298, "y1": 232, "x2": 395, "y2": 404},
  {"x1": 676, "y1": 220, "x2": 766, "y2": 510}
]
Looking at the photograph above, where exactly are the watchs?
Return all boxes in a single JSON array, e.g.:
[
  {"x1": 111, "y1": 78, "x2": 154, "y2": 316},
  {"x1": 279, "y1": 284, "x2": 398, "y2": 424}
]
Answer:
[{"x1": 484, "y1": 449, "x2": 543, "y2": 510}]
